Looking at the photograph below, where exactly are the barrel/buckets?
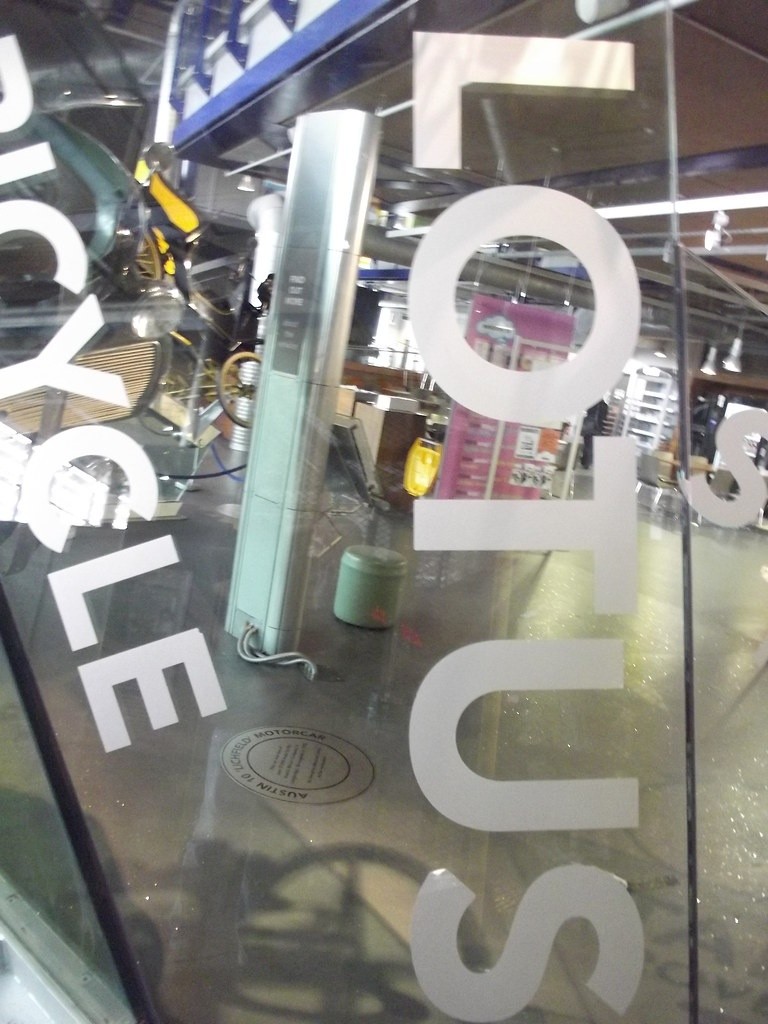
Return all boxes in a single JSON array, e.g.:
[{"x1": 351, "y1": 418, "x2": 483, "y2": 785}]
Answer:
[{"x1": 228, "y1": 361, "x2": 260, "y2": 451}]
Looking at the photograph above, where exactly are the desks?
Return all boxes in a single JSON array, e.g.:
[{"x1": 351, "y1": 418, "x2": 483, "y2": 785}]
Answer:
[{"x1": 655, "y1": 456, "x2": 718, "y2": 494}]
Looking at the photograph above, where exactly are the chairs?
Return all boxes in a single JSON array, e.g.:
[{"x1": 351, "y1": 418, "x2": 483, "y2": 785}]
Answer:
[{"x1": 636, "y1": 446, "x2": 735, "y2": 525}]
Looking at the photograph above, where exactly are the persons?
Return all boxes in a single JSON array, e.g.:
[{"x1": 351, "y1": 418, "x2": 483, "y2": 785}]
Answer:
[
  {"x1": 256, "y1": 274, "x2": 277, "y2": 310},
  {"x1": 579, "y1": 397, "x2": 610, "y2": 468}
]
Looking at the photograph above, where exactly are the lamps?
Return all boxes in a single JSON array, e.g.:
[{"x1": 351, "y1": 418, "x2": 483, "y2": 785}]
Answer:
[
  {"x1": 723, "y1": 289, "x2": 755, "y2": 373},
  {"x1": 381, "y1": 212, "x2": 413, "y2": 232},
  {"x1": 705, "y1": 210, "x2": 732, "y2": 251},
  {"x1": 235, "y1": 175, "x2": 257, "y2": 192},
  {"x1": 700, "y1": 304, "x2": 735, "y2": 377}
]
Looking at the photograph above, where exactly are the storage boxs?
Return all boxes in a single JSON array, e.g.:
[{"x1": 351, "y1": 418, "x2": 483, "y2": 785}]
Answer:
[
  {"x1": 337, "y1": 385, "x2": 380, "y2": 415},
  {"x1": 353, "y1": 400, "x2": 427, "y2": 489},
  {"x1": 377, "y1": 395, "x2": 418, "y2": 411}
]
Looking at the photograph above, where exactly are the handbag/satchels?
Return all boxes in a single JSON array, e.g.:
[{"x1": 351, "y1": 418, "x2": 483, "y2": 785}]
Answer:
[{"x1": 579, "y1": 416, "x2": 604, "y2": 437}]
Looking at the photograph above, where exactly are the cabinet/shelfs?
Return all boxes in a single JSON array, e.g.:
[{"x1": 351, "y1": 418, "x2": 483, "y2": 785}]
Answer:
[{"x1": 619, "y1": 373, "x2": 678, "y2": 448}]
